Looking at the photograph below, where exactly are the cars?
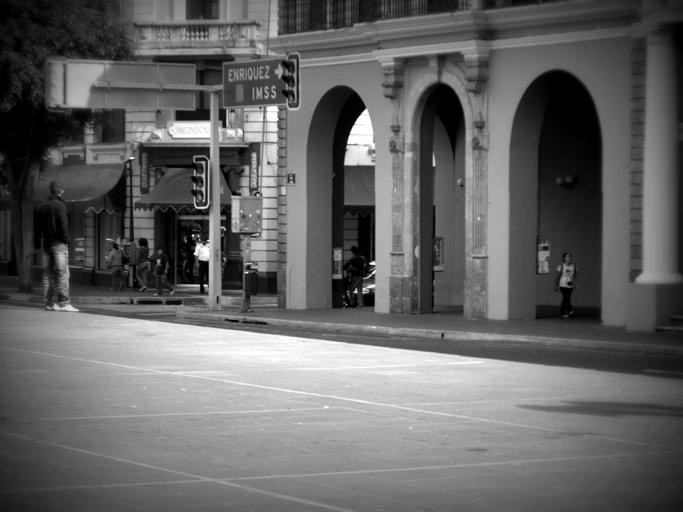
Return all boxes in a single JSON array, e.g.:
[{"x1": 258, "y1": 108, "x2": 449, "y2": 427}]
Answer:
[{"x1": 347, "y1": 260, "x2": 375, "y2": 307}]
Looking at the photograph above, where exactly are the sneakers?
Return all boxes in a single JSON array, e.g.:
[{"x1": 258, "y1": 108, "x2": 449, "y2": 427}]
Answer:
[
  {"x1": 60, "y1": 305, "x2": 80, "y2": 312},
  {"x1": 46, "y1": 303, "x2": 60, "y2": 310}
]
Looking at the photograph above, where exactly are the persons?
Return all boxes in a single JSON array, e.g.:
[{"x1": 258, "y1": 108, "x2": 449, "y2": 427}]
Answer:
[
  {"x1": 135, "y1": 238, "x2": 150, "y2": 293},
  {"x1": 149, "y1": 248, "x2": 175, "y2": 295},
  {"x1": 194, "y1": 237, "x2": 209, "y2": 295},
  {"x1": 33, "y1": 180, "x2": 78, "y2": 311},
  {"x1": 108, "y1": 243, "x2": 126, "y2": 292},
  {"x1": 554, "y1": 252, "x2": 578, "y2": 319},
  {"x1": 344, "y1": 246, "x2": 365, "y2": 308},
  {"x1": 342, "y1": 270, "x2": 351, "y2": 308}
]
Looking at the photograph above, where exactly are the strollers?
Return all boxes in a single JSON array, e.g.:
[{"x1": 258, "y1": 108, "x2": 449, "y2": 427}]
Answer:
[{"x1": 342, "y1": 264, "x2": 350, "y2": 308}]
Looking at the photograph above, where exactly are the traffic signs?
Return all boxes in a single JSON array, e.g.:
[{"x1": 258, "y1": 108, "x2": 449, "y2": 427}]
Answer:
[{"x1": 221, "y1": 57, "x2": 288, "y2": 109}]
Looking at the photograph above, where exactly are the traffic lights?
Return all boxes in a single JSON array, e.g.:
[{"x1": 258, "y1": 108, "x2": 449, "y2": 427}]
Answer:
[
  {"x1": 191, "y1": 155, "x2": 210, "y2": 210},
  {"x1": 281, "y1": 52, "x2": 301, "y2": 111}
]
